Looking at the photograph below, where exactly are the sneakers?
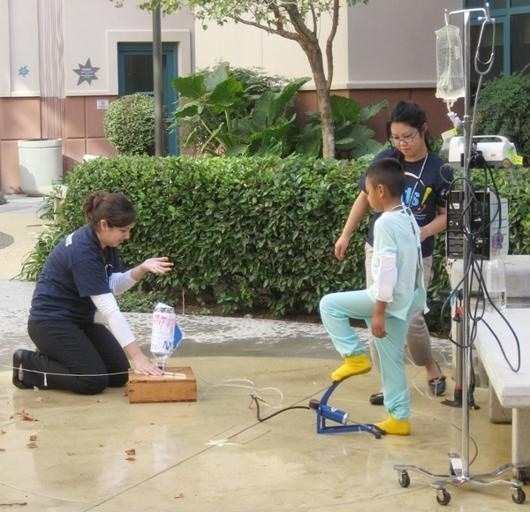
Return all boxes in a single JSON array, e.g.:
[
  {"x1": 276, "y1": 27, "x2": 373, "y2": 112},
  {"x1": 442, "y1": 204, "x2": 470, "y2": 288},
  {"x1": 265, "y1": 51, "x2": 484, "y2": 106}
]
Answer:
[
  {"x1": 328, "y1": 352, "x2": 374, "y2": 383},
  {"x1": 375, "y1": 414, "x2": 411, "y2": 436}
]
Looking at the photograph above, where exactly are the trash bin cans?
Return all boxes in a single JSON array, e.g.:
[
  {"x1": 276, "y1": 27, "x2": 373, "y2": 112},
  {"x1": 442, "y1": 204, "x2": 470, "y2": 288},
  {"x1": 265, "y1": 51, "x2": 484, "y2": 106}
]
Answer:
[
  {"x1": 18, "y1": 139, "x2": 62, "y2": 197},
  {"x1": 447, "y1": 134, "x2": 520, "y2": 170}
]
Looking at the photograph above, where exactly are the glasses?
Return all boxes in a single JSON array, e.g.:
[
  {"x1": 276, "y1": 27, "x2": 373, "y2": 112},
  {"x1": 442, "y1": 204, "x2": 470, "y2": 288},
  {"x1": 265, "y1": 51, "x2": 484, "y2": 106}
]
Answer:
[{"x1": 388, "y1": 126, "x2": 422, "y2": 144}]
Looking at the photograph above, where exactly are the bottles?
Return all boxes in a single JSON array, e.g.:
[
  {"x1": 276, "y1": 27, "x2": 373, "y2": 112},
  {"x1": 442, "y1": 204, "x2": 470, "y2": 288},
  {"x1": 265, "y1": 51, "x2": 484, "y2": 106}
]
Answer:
[
  {"x1": 151, "y1": 306, "x2": 175, "y2": 372},
  {"x1": 482, "y1": 239, "x2": 507, "y2": 313}
]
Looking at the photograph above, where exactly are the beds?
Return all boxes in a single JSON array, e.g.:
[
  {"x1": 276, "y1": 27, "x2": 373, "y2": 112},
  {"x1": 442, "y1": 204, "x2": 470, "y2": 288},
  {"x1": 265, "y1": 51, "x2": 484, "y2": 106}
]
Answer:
[{"x1": 448, "y1": 254, "x2": 529, "y2": 478}]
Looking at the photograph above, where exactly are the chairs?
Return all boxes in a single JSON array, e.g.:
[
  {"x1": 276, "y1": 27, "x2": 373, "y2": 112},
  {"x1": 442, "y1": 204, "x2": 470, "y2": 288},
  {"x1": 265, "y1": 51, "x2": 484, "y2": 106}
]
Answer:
[{"x1": 37, "y1": 155, "x2": 110, "y2": 223}]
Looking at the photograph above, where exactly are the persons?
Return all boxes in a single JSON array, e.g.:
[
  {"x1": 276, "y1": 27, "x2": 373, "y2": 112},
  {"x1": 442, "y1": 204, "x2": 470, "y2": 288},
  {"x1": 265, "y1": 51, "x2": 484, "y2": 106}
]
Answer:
[
  {"x1": 318, "y1": 158, "x2": 429, "y2": 436},
  {"x1": 334, "y1": 99, "x2": 456, "y2": 405},
  {"x1": 12, "y1": 190, "x2": 175, "y2": 394}
]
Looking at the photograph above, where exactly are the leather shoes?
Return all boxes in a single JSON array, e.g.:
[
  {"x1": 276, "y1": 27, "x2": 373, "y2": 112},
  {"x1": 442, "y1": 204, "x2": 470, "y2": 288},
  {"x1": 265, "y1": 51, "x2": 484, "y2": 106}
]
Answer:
[
  {"x1": 12, "y1": 347, "x2": 28, "y2": 391},
  {"x1": 424, "y1": 358, "x2": 448, "y2": 396},
  {"x1": 367, "y1": 391, "x2": 386, "y2": 405}
]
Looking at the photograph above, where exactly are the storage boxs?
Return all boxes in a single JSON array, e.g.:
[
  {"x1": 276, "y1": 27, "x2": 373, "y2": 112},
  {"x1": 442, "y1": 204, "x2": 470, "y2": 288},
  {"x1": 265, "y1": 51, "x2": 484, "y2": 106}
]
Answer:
[{"x1": 128, "y1": 366, "x2": 198, "y2": 404}]
